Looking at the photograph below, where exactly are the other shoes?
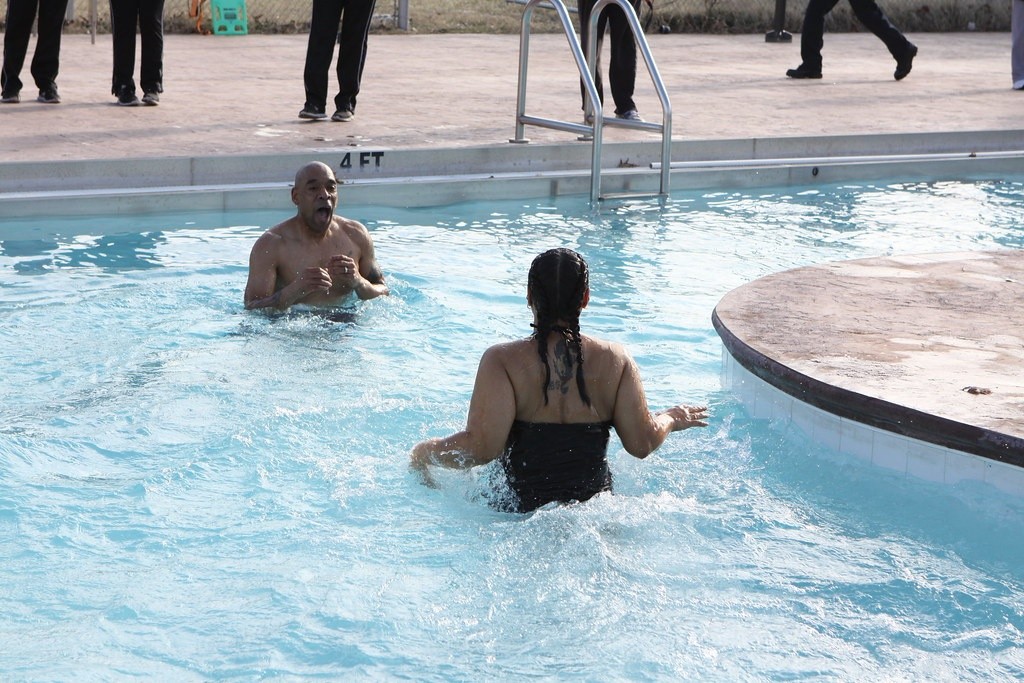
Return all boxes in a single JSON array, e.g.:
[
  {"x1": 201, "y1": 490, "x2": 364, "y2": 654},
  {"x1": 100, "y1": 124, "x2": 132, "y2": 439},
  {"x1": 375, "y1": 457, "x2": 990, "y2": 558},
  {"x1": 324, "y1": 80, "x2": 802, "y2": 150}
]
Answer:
[
  {"x1": 894, "y1": 44, "x2": 918, "y2": 80},
  {"x1": 786, "y1": 64, "x2": 823, "y2": 79}
]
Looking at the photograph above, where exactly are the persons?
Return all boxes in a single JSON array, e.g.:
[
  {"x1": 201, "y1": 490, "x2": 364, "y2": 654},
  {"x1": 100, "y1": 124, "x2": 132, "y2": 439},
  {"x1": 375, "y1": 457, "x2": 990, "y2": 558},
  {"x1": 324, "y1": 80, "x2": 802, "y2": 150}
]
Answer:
[
  {"x1": 243, "y1": 161, "x2": 390, "y2": 313},
  {"x1": 298, "y1": 0, "x2": 376, "y2": 122},
  {"x1": 785, "y1": 0, "x2": 918, "y2": 81},
  {"x1": 436, "y1": 248, "x2": 711, "y2": 513},
  {"x1": 578, "y1": 0, "x2": 645, "y2": 122},
  {"x1": 1, "y1": 0, "x2": 68, "y2": 103},
  {"x1": 109, "y1": 0, "x2": 165, "y2": 106}
]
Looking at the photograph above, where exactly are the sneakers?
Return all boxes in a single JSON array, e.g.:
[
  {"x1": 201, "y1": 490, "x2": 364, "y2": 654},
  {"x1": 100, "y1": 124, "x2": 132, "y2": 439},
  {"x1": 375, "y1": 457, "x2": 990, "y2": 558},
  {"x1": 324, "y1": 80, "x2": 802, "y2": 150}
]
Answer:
[
  {"x1": 117, "y1": 94, "x2": 139, "y2": 106},
  {"x1": 2, "y1": 94, "x2": 19, "y2": 103},
  {"x1": 332, "y1": 108, "x2": 354, "y2": 122},
  {"x1": 299, "y1": 103, "x2": 327, "y2": 119},
  {"x1": 38, "y1": 87, "x2": 61, "y2": 104},
  {"x1": 142, "y1": 92, "x2": 159, "y2": 104},
  {"x1": 616, "y1": 110, "x2": 645, "y2": 123}
]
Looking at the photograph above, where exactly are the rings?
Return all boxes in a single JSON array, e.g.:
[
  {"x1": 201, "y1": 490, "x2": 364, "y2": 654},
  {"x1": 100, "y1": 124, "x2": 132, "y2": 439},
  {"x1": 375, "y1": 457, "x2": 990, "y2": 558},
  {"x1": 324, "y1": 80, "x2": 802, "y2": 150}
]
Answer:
[{"x1": 344, "y1": 267, "x2": 348, "y2": 274}]
